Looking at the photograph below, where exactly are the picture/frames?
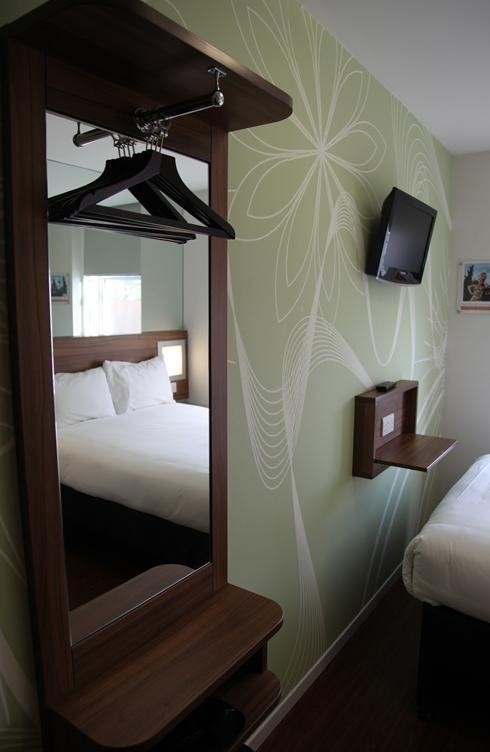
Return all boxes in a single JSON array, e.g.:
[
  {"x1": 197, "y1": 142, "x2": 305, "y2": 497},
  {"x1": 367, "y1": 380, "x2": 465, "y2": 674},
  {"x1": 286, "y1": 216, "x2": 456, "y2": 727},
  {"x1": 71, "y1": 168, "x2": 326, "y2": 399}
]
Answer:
[{"x1": 51, "y1": 272, "x2": 70, "y2": 302}]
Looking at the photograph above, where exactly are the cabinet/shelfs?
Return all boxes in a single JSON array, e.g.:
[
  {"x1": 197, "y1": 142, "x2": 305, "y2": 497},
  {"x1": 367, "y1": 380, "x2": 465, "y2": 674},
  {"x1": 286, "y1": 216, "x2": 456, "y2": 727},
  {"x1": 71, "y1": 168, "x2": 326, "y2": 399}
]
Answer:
[{"x1": 39, "y1": 583, "x2": 285, "y2": 751}]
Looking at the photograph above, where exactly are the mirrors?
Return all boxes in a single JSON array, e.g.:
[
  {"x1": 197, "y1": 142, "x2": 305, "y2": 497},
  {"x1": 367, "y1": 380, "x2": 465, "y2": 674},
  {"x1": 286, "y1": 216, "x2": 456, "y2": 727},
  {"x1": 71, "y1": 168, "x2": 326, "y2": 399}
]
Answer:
[{"x1": 0, "y1": 0, "x2": 292, "y2": 699}]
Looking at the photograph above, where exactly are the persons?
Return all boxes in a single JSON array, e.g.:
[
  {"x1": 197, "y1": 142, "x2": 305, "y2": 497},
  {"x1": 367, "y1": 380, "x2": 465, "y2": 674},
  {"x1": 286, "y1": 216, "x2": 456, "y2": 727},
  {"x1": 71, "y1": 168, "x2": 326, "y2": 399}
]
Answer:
[{"x1": 467, "y1": 272, "x2": 490, "y2": 301}]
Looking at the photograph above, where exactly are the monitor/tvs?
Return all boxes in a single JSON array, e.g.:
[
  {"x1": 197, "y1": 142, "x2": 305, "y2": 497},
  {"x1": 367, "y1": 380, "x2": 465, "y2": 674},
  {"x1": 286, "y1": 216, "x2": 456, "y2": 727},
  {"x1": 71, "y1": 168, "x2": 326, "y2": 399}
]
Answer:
[{"x1": 367, "y1": 187, "x2": 437, "y2": 287}]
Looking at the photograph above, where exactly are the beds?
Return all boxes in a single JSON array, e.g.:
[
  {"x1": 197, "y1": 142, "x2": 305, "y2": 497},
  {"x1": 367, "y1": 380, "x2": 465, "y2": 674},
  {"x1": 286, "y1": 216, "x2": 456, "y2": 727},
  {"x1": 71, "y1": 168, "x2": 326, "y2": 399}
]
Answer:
[
  {"x1": 397, "y1": 455, "x2": 489, "y2": 615},
  {"x1": 51, "y1": 334, "x2": 211, "y2": 535}
]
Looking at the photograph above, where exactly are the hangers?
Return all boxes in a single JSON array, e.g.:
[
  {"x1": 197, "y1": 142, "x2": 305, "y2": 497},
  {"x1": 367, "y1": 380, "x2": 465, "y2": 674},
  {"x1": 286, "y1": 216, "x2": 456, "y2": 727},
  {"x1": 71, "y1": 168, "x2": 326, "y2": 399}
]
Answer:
[
  {"x1": 46, "y1": 133, "x2": 196, "y2": 243},
  {"x1": 58, "y1": 102, "x2": 239, "y2": 239}
]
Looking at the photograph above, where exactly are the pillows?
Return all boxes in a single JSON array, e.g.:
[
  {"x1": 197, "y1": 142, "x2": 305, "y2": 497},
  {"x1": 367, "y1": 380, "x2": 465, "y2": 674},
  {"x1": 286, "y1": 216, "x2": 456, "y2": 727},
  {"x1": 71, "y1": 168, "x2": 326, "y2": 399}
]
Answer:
[{"x1": 53, "y1": 353, "x2": 176, "y2": 430}]
